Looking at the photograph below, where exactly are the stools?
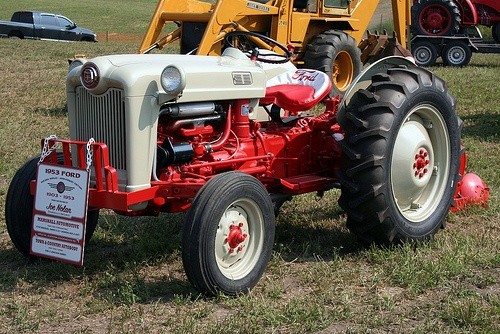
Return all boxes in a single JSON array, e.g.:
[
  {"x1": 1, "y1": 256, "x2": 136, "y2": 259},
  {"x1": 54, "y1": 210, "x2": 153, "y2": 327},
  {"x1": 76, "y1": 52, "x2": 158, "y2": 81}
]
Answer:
[{"x1": 256, "y1": 68, "x2": 332, "y2": 124}]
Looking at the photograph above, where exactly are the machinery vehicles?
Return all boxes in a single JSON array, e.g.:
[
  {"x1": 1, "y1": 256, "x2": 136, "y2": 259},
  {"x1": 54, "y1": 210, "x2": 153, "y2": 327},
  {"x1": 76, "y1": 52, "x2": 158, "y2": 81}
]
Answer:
[
  {"x1": 134, "y1": 0, "x2": 414, "y2": 92},
  {"x1": 406, "y1": 0, "x2": 500, "y2": 68},
  {"x1": 8, "y1": 27, "x2": 463, "y2": 300}
]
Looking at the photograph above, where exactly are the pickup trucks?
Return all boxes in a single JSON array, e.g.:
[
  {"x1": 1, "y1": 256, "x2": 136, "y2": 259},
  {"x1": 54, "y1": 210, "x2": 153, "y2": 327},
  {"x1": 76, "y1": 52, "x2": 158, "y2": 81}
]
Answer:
[{"x1": 0, "y1": 9, "x2": 98, "y2": 44}]
